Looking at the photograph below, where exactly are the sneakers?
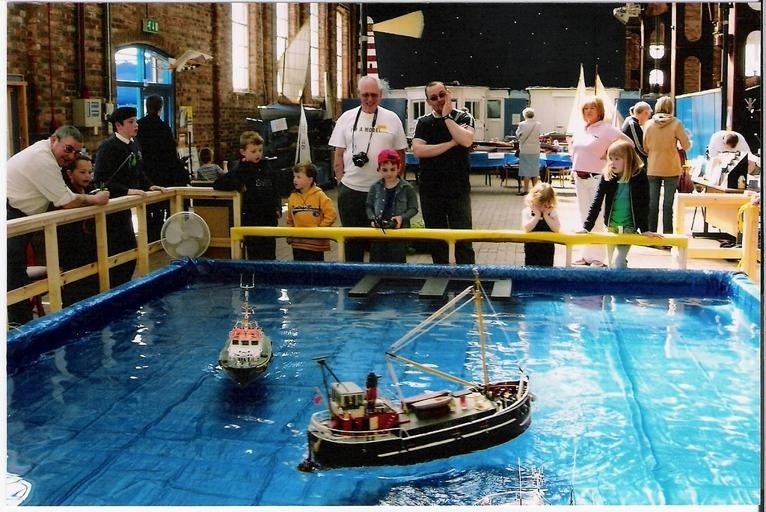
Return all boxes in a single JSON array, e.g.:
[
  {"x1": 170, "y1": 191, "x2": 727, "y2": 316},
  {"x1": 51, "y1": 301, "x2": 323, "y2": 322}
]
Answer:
[
  {"x1": 515, "y1": 191, "x2": 528, "y2": 196},
  {"x1": 571, "y1": 258, "x2": 587, "y2": 264},
  {"x1": 590, "y1": 260, "x2": 604, "y2": 267}
]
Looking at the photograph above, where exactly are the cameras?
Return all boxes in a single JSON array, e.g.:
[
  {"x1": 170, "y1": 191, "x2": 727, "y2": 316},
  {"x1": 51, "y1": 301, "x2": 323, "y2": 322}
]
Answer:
[{"x1": 352, "y1": 153, "x2": 369, "y2": 167}]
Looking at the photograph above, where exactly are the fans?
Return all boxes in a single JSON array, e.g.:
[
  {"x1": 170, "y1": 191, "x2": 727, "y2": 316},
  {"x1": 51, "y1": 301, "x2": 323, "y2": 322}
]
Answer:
[{"x1": 160, "y1": 211, "x2": 212, "y2": 260}]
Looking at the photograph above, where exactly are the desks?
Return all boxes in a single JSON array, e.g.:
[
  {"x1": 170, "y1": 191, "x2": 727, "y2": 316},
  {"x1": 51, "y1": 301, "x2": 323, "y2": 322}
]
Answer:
[
  {"x1": 690, "y1": 174, "x2": 761, "y2": 246},
  {"x1": 405, "y1": 149, "x2": 572, "y2": 186}
]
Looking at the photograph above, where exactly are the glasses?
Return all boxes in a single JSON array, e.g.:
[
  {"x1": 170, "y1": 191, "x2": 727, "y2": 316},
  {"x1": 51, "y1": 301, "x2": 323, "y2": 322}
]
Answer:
[
  {"x1": 360, "y1": 93, "x2": 378, "y2": 97},
  {"x1": 426, "y1": 92, "x2": 446, "y2": 101},
  {"x1": 57, "y1": 134, "x2": 82, "y2": 160}
]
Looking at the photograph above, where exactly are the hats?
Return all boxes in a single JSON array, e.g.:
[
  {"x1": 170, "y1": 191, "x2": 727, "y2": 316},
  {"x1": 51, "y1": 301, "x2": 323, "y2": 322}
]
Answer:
[
  {"x1": 378, "y1": 149, "x2": 401, "y2": 164},
  {"x1": 109, "y1": 108, "x2": 137, "y2": 125}
]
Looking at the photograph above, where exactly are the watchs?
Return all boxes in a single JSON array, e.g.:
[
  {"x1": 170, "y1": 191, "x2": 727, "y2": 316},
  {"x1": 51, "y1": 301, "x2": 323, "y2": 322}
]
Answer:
[{"x1": 443, "y1": 113, "x2": 454, "y2": 120}]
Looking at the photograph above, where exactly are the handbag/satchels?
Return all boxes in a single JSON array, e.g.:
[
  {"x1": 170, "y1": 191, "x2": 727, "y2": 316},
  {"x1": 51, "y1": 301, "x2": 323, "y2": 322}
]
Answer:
[{"x1": 514, "y1": 149, "x2": 520, "y2": 159}]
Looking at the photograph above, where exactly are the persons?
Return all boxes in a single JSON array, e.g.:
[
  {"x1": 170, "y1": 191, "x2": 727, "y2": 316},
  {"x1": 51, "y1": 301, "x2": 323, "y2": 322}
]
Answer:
[
  {"x1": 135, "y1": 94, "x2": 179, "y2": 242},
  {"x1": 514, "y1": 107, "x2": 542, "y2": 196},
  {"x1": 621, "y1": 101, "x2": 649, "y2": 173},
  {"x1": 45, "y1": 156, "x2": 96, "y2": 307},
  {"x1": 326, "y1": 76, "x2": 408, "y2": 263},
  {"x1": 92, "y1": 106, "x2": 167, "y2": 287},
  {"x1": 285, "y1": 161, "x2": 337, "y2": 262},
  {"x1": 195, "y1": 147, "x2": 229, "y2": 182},
  {"x1": 643, "y1": 95, "x2": 692, "y2": 235},
  {"x1": 365, "y1": 148, "x2": 419, "y2": 262},
  {"x1": 212, "y1": 130, "x2": 282, "y2": 259},
  {"x1": 570, "y1": 96, "x2": 635, "y2": 266},
  {"x1": 7, "y1": 124, "x2": 111, "y2": 329},
  {"x1": 705, "y1": 129, "x2": 750, "y2": 162},
  {"x1": 576, "y1": 139, "x2": 664, "y2": 269},
  {"x1": 412, "y1": 80, "x2": 476, "y2": 265},
  {"x1": 520, "y1": 181, "x2": 561, "y2": 266}
]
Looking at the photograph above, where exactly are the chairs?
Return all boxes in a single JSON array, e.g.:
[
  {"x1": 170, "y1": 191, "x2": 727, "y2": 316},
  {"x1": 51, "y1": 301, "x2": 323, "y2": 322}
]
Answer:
[
  {"x1": 505, "y1": 163, "x2": 525, "y2": 190},
  {"x1": 544, "y1": 167, "x2": 566, "y2": 189}
]
